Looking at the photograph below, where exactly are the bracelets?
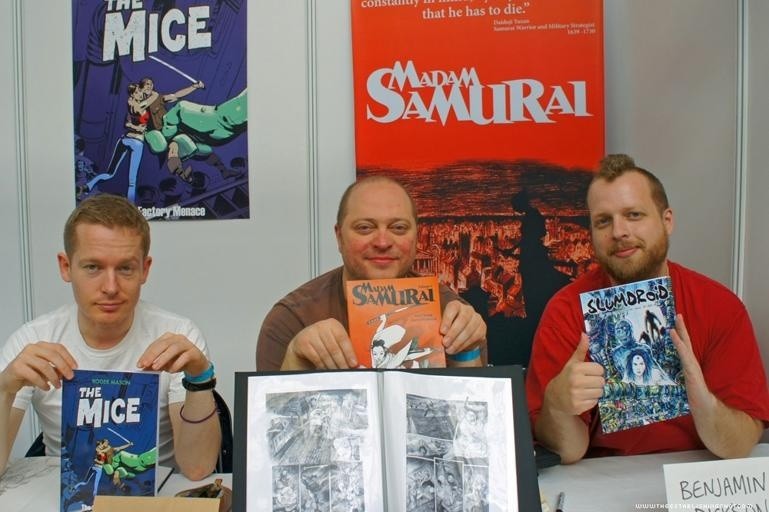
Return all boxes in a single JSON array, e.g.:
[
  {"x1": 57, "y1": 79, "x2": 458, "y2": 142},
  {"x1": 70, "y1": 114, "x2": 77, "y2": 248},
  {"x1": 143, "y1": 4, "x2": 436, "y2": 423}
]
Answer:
[
  {"x1": 184, "y1": 361, "x2": 215, "y2": 383},
  {"x1": 179, "y1": 402, "x2": 218, "y2": 422},
  {"x1": 181, "y1": 377, "x2": 218, "y2": 392},
  {"x1": 444, "y1": 347, "x2": 481, "y2": 363}
]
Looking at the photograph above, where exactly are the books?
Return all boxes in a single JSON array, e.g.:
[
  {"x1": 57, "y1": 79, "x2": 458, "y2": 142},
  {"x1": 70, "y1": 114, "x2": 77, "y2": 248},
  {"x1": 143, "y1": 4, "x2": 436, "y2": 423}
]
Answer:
[
  {"x1": 59, "y1": 365, "x2": 165, "y2": 512},
  {"x1": 579, "y1": 277, "x2": 692, "y2": 437},
  {"x1": 347, "y1": 271, "x2": 449, "y2": 370},
  {"x1": 232, "y1": 365, "x2": 543, "y2": 512}
]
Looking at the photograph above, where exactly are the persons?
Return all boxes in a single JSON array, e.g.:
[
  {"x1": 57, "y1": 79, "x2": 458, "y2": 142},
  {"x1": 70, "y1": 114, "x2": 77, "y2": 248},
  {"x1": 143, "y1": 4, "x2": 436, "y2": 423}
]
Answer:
[
  {"x1": 0, "y1": 192, "x2": 225, "y2": 484},
  {"x1": 523, "y1": 152, "x2": 769, "y2": 465},
  {"x1": 75, "y1": 160, "x2": 97, "y2": 201},
  {"x1": 255, "y1": 176, "x2": 489, "y2": 370},
  {"x1": 74, "y1": 134, "x2": 86, "y2": 165}
]
